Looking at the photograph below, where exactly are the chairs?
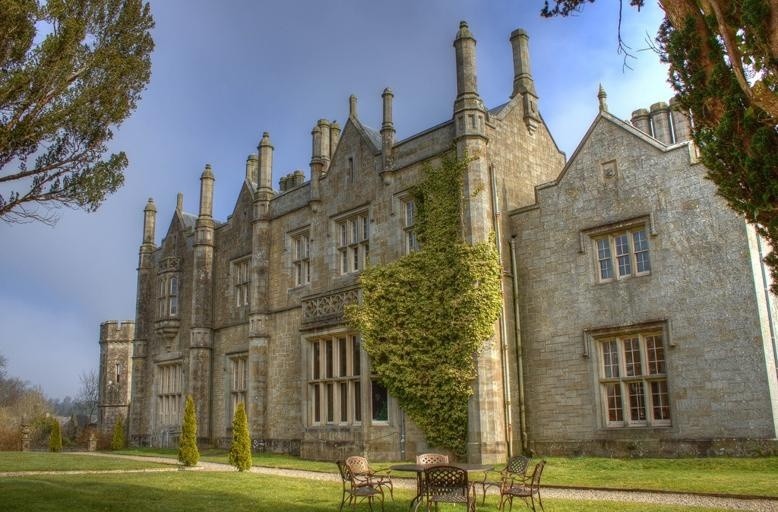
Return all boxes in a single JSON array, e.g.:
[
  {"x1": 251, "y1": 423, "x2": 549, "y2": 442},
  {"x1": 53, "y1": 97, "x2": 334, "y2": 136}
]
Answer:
[
  {"x1": 337, "y1": 456, "x2": 395, "y2": 512},
  {"x1": 482, "y1": 456, "x2": 529, "y2": 506},
  {"x1": 423, "y1": 466, "x2": 476, "y2": 511},
  {"x1": 501, "y1": 459, "x2": 547, "y2": 512},
  {"x1": 415, "y1": 454, "x2": 449, "y2": 502}
]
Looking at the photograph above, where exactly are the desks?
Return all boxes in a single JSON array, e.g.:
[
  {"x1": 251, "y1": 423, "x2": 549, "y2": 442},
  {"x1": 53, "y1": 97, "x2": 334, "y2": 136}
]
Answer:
[{"x1": 390, "y1": 464, "x2": 495, "y2": 512}]
